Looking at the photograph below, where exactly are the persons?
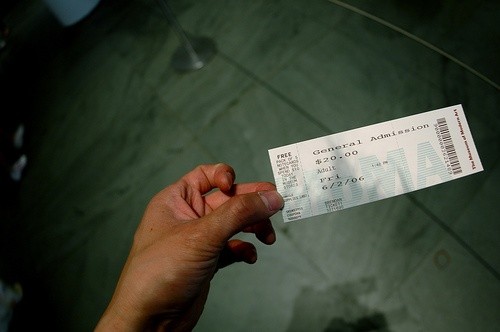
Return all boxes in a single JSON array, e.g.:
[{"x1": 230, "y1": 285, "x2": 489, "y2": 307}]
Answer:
[{"x1": 91, "y1": 161, "x2": 285, "y2": 332}]
